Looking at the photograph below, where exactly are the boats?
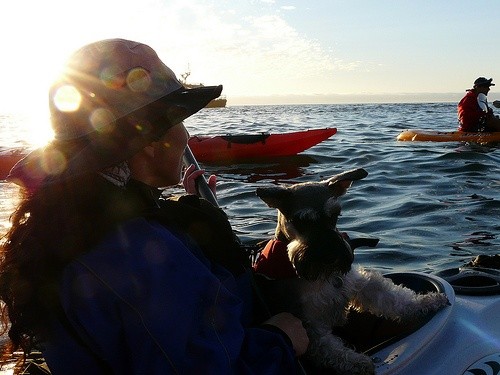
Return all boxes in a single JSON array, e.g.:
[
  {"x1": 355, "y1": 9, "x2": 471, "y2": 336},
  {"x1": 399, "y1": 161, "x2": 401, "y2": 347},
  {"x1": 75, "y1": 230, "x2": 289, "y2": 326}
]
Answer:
[
  {"x1": 397, "y1": 127, "x2": 500, "y2": 144},
  {"x1": 179, "y1": 63, "x2": 228, "y2": 108},
  {"x1": 0, "y1": 127, "x2": 338, "y2": 181}
]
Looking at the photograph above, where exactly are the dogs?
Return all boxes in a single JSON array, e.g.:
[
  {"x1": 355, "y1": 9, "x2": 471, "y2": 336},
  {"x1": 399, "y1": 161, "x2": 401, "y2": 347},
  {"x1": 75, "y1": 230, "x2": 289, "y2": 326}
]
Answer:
[{"x1": 252, "y1": 167, "x2": 453, "y2": 375}]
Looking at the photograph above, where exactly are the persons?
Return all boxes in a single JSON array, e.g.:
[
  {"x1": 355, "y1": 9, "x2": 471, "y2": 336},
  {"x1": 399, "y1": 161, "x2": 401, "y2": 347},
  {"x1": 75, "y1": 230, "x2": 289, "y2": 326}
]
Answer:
[
  {"x1": 458, "y1": 77, "x2": 500, "y2": 133},
  {"x1": 0, "y1": 38, "x2": 310, "y2": 375}
]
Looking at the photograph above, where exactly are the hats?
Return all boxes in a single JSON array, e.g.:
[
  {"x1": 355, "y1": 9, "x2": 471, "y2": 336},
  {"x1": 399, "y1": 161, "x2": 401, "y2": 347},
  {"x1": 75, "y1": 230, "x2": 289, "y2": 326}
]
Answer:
[
  {"x1": 474, "y1": 77, "x2": 495, "y2": 86},
  {"x1": 6, "y1": 38, "x2": 223, "y2": 186}
]
